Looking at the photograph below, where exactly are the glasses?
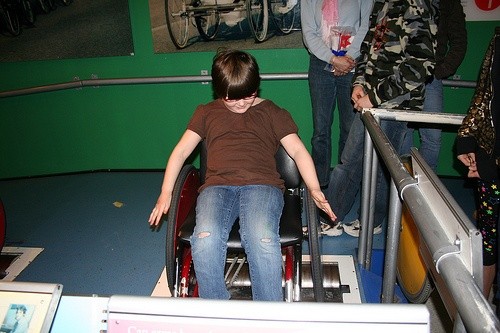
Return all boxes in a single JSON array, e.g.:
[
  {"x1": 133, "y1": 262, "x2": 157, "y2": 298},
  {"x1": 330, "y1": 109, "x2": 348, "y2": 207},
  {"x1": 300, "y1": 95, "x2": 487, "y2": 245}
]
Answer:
[{"x1": 223, "y1": 91, "x2": 256, "y2": 102}]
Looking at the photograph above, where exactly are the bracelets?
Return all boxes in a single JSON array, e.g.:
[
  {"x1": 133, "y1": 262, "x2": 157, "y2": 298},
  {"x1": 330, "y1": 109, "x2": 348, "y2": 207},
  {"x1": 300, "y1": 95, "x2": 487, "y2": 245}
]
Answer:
[{"x1": 333, "y1": 56, "x2": 336, "y2": 65}]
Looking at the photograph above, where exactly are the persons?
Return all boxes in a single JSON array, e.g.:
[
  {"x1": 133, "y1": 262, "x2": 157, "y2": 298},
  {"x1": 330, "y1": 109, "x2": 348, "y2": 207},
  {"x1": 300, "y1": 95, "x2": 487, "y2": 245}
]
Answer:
[
  {"x1": 300, "y1": 0, "x2": 375, "y2": 191},
  {"x1": 148, "y1": 47, "x2": 337, "y2": 302},
  {"x1": 400, "y1": 0, "x2": 467, "y2": 176},
  {"x1": 455, "y1": 27, "x2": 500, "y2": 322},
  {"x1": 303, "y1": 0, "x2": 439, "y2": 237}
]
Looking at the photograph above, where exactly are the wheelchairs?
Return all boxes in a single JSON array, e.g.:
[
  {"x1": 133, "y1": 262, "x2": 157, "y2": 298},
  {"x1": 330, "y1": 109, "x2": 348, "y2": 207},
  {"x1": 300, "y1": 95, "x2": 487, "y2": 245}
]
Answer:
[{"x1": 165, "y1": 133, "x2": 326, "y2": 302}]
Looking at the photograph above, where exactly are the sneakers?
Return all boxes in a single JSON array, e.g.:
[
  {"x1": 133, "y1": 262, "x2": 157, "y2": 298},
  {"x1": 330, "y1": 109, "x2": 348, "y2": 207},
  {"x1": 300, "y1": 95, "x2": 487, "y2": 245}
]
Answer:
[
  {"x1": 302, "y1": 221, "x2": 342, "y2": 236},
  {"x1": 344, "y1": 219, "x2": 382, "y2": 237}
]
